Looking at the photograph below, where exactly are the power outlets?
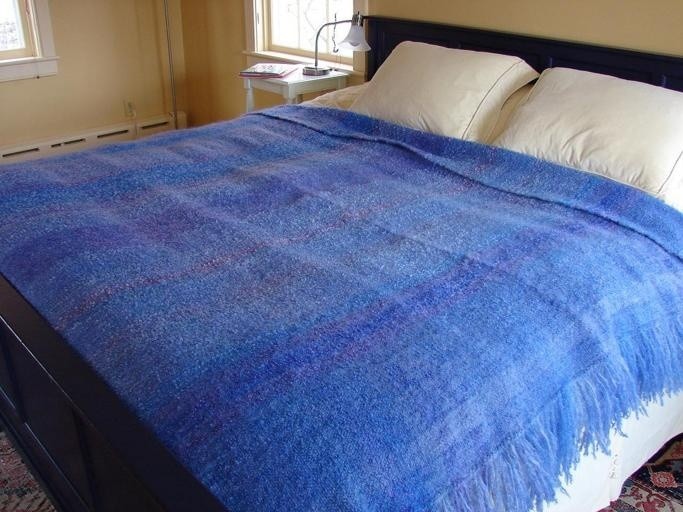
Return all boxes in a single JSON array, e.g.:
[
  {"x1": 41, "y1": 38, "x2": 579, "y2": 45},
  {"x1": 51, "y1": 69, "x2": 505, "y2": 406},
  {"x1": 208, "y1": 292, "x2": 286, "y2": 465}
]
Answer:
[{"x1": 124, "y1": 97, "x2": 136, "y2": 119}]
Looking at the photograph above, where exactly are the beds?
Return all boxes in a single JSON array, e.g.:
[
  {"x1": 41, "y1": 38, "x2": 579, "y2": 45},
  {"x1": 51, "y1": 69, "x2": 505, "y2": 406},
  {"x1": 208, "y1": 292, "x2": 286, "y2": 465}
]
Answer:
[{"x1": 0, "y1": 16, "x2": 683, "y2": 512}]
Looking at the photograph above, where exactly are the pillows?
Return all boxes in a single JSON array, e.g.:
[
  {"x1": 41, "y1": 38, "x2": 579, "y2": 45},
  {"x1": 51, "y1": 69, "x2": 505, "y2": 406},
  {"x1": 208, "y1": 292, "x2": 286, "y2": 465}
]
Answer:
[
  {"x1": 349, "y1": 41, "x2": 538, "y2": 144},
  {"x1": 491, "y1": 67, "x2": 683, "y2": 197}
]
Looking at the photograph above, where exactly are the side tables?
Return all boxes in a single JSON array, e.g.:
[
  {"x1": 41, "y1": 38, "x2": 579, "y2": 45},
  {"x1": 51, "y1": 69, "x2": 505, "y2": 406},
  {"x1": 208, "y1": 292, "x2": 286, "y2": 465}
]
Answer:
[{"x1": 244, "y1": 63, "x2": 349, "y2": 114}]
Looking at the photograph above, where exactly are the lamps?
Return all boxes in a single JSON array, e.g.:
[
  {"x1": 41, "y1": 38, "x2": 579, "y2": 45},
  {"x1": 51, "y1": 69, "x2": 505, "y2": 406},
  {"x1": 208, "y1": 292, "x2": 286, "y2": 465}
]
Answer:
[{"x1": 303, "y1": 12, "x2": 371, "y2": 77}]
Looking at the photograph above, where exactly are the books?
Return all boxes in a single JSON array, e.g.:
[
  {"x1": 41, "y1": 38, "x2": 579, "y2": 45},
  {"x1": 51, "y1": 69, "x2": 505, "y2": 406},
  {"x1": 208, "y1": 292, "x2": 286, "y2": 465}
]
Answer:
[{"x1": 239, "y1": 63, "x2": 300, "y2": 78}]
